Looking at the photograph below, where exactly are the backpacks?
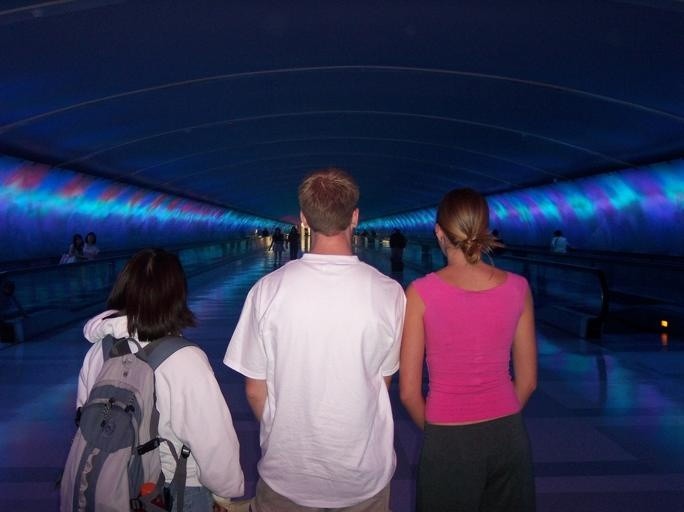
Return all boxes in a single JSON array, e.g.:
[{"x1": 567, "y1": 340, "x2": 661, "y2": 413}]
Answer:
[{"x1": 57, "y1": 334, "x2": 205, "y2": 512}]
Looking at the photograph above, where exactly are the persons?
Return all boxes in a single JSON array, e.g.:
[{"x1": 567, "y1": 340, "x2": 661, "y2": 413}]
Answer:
[
  {"x1": 224, "y1": 169, "x2": 407, "y2": 511},
  {"x1": 390, "y1": 229, "x2": 406, "y2": 264},
  {"x1": 399, "y1": 186, "x2": 537, "y2": 511},
  {"x1": 550, "y1": 230, "x2": 577, "y2": 256},
  {"x1": 269, "y1": 226, "x2": 300, "y2": 266},
  {"x1": 489, "y1": 229, "x2": 503, "y2": 257},
  {"x1": 69, "y1": 232, "x2": 100, "y2": 260},
  {"x1": 76, "y1": 247, "x2": 245, "y2": 510}
]
